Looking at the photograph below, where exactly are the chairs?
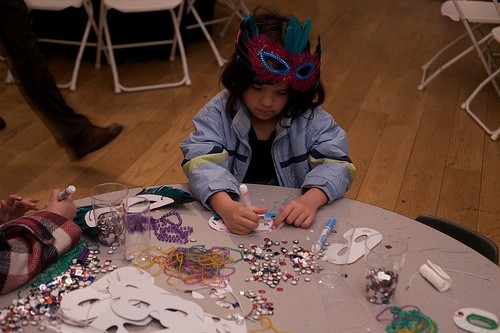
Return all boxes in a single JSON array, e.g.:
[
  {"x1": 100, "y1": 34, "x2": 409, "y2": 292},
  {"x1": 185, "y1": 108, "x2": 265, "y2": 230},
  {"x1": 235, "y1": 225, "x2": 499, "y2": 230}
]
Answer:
[
  {"x1": 460, "y1": 27, "x2": 500, "y2": 141},
  {"x1": 417, "y1": 0, "x2": 500, "y2": 91},
  {"x1": 186, "y1": 0, "x2": 251, "y2": 67},
  {"x1": 414, "y1": 214, "x2": 499, "y2": 266},
  {"x1": 95, "y1": 0, "x2": 192, "y2": 94},
  {"x1": 6, "y1": 0, "x2": 111, "y2": 92}
]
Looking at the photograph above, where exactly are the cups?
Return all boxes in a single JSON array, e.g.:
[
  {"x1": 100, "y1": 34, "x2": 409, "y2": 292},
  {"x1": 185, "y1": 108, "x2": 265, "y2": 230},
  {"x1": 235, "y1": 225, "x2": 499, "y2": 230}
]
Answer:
[
  {"x1": 113, "y1": 196, "x2": 150, "y2": 261},
  {"x1": 312, "y1": 217, "x2": 354, "y2": 287},
  {"x1": 364, "y1": 233, "x2": 408, "y2": 305},
  {"x1": 89, "y1": 183, "x2": 129, "y2": 247}
]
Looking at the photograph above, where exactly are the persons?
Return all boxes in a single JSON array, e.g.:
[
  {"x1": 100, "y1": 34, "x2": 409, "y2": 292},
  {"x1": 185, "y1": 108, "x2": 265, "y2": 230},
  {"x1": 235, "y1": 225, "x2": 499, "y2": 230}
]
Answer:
[
  {"x1": 178, "y1": 5, "x2": 356, "y2": 235},
  {"x1": 0, "y1": 117, "x2": 81, "y2": 296},
  {"x1": 0, "y1": 0, "x2": 125, "y2": 161}
]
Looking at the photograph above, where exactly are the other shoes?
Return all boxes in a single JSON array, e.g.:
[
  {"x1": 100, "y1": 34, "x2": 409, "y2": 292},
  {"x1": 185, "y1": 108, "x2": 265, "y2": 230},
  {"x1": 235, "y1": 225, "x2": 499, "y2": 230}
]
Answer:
[{"x1": 64, "y1": 122, "x2": 123, "y2": 162}]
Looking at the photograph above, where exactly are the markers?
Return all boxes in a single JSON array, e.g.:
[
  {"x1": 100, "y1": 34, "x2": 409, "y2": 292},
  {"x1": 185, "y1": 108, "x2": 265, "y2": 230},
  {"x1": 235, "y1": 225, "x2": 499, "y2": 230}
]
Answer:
[
  {"x1": 58, "y1": 185, "x2": 76, "y2": 200},
  {"x1": 311, "y1": 216, "x2": 336, "y2": 253},
  {"x1": 240, "y1": 184, "x2": 253, "y2": 212}
]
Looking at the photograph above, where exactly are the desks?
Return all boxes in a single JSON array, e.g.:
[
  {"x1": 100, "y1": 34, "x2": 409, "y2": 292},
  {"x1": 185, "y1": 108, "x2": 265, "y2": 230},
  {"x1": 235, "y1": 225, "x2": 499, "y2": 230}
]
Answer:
[{"x1": 0, "y1": 183, "x2": 500, "y2": 333}]
